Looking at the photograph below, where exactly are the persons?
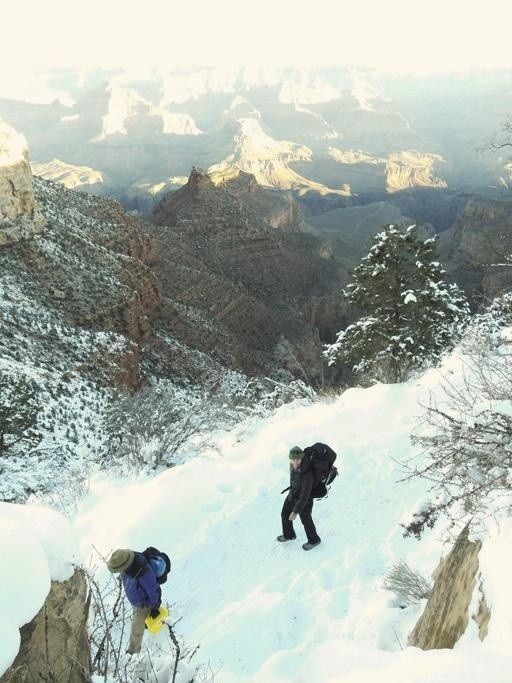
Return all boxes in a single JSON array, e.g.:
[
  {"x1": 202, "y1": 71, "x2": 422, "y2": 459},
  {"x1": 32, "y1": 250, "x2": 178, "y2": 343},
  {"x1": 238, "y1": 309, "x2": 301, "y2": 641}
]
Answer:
[
  {"x1": 107, "y1": 549, "x2": 162, "y2": 661},
  {"x1": 276, "y1": 446, "x2": 321, "y2": 551}
]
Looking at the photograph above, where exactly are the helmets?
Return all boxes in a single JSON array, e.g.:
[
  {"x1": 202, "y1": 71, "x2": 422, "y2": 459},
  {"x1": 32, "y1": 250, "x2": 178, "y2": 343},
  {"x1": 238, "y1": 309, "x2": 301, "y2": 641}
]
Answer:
[{"x1": 105, "y1": 548, "x2": 135, "y2": 574}]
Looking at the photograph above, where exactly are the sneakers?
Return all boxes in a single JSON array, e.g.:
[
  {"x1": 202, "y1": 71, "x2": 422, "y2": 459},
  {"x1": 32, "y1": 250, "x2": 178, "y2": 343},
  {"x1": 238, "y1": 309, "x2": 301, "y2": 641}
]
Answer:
[
  {"x1": 276, "y1": 533, "x2": 296, "y2": 542},
  {"x1": 301, "y1": 539, "x2": 321, "y2": 551}
]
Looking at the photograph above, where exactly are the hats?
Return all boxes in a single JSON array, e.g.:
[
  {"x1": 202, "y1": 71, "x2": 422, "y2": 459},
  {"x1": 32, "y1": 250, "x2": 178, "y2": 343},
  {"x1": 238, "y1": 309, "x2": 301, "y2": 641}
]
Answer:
[{"x1": 289, "y1": 446, "x2": 305, "y2": 460}]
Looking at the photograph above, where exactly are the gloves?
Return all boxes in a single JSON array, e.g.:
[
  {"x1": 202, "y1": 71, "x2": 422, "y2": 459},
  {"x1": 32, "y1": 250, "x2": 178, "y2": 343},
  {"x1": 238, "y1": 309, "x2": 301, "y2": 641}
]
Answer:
[{"x1": 149, "y1": 607, "x2": 160, "y2": 619}]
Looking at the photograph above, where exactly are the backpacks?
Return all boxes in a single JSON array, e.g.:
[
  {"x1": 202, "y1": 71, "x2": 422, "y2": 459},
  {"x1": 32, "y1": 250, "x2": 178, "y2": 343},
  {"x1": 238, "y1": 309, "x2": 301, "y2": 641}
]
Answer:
[
  {"x1": 141, "y1": 546, "x2": 170, "y2": 585},
  {"x1": 302, "y1": 441, "x2": 340, "y2": 499}
]
